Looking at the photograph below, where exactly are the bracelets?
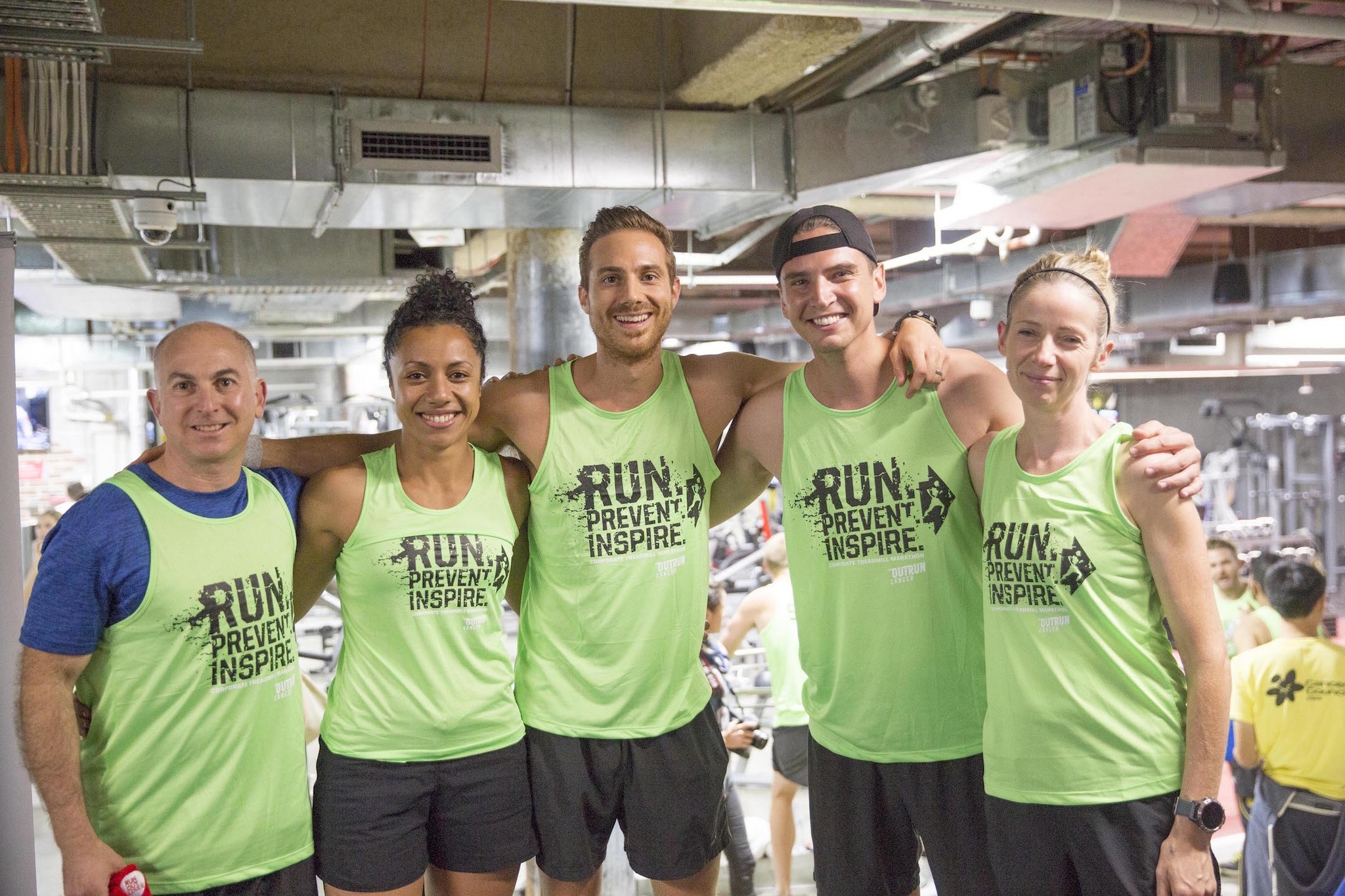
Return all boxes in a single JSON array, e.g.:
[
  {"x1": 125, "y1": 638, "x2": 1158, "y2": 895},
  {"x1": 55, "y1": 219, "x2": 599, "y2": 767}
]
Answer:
[{"x1": 242, "y1": 434, "x2": 261, "y2": 470}]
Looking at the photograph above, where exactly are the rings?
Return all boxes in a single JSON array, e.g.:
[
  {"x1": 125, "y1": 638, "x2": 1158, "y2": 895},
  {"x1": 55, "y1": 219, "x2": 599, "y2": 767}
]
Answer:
[{"x1": 935, "y1": 370, "x2": 943, "y2": 376}]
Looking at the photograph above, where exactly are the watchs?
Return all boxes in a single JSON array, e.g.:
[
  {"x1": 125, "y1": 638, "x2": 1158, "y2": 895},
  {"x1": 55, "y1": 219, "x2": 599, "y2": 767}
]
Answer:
[
  {"x1": 892, "y1": 309, "x2": 937, "y2": 341},
  {"x1": 1172, "y1": 795, "x2": 1225, "y2": 833}
]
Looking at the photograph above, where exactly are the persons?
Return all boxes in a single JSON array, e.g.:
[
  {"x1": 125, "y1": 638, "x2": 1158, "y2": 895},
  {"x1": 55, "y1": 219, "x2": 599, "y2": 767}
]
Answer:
[
  {"x1": 17, "y1": 325, "x2": 527, "y2": 896},
  {"x1": 22, "y1": 481, "x2": 88, "y2": 603},
  {"x1": 125, "y1": 204, "x2": 952, "y2": 896},
  {"x1": 1205, "y1": 537, "x2": 1345, "y2": 896},
  {"x1": 696, "y1": 205, "x2": 1203, "y2": 896},
  {"x1": 965, "y1": 243, "x2": 1231, "y2": 896},
  {"x1": 293, "y1": 267, "x2": 585, "y2": 896},
  {"x1": 697, "y1": 533, "x2": 820, "y2": 896}
]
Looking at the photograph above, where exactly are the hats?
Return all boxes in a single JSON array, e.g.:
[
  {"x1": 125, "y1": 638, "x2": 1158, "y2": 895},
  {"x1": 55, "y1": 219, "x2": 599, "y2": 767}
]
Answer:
[{"x1": 772, "y1": 205, "x2": 879, "y2": 317}]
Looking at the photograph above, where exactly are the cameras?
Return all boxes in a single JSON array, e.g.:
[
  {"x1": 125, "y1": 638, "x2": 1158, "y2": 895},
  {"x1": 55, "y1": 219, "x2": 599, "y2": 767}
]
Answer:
[{"x1": 728, "y1": 710, "x2": 768, "y2": 756}]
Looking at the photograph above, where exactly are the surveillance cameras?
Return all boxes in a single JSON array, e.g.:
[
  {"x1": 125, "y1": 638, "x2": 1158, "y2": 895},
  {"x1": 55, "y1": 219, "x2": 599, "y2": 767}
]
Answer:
[{"x1": 133, "y1": 211, "x2": 178, "y2": 247}]
